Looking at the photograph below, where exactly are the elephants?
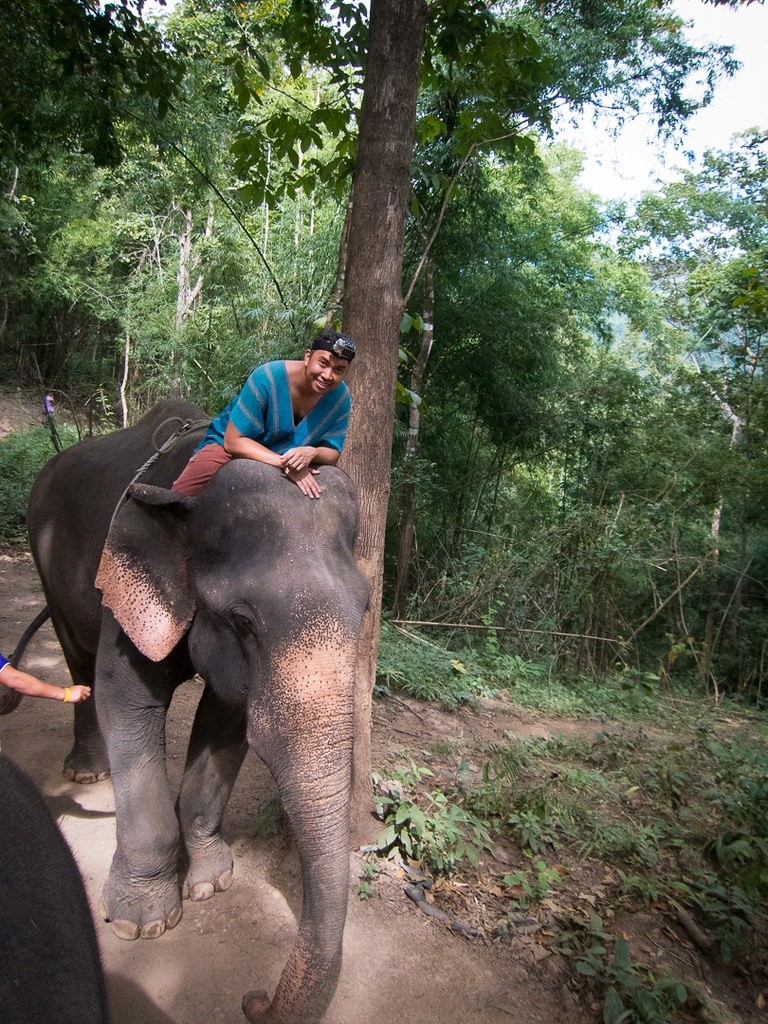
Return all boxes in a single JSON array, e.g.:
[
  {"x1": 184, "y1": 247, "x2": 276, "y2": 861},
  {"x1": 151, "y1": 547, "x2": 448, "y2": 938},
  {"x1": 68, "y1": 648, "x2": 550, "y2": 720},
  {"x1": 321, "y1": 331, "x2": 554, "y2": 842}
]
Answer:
[{"x1": 1, "y1": 398, "x2": 374, "y2": 1024}]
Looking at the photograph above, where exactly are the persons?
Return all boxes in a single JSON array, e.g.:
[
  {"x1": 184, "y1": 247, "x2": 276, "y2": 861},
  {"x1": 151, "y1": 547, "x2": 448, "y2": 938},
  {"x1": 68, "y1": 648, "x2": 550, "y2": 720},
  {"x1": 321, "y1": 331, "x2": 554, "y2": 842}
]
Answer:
[
  {"x1": 44, "y1": 390, "x2": 55, "y2": 429},
  {"x1": 171, "y1": 330, "x2": 356, "y2": 499},
  {"x1": 0, "y1": 652, "x2": 91, "y2": 703}
]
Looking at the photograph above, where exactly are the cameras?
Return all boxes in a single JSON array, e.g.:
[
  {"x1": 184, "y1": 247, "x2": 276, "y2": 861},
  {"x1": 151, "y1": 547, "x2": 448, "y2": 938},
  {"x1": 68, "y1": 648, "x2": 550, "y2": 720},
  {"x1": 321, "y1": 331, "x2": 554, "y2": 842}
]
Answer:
[{"x1": 330, "y1": 337, "x2": 356, "y2": 359}]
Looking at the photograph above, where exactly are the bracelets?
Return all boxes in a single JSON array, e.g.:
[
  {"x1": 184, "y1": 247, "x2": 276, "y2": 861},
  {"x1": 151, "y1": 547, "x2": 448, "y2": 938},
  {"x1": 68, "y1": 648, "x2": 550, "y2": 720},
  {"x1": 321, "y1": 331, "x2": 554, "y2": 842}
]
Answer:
[{"x1": 62, "y1": 687, "x2": 70, "y2": 703}]
